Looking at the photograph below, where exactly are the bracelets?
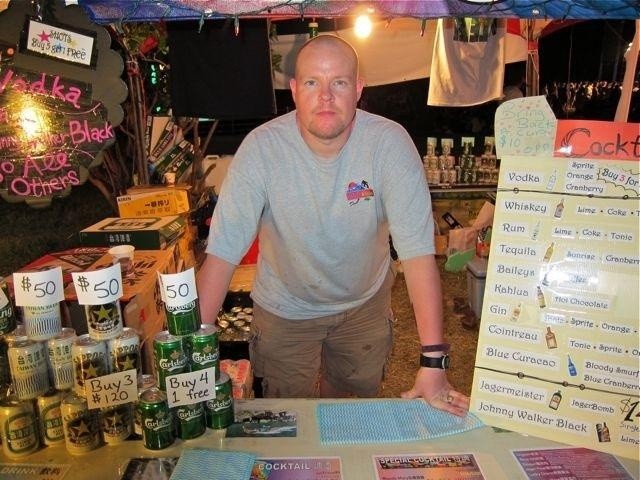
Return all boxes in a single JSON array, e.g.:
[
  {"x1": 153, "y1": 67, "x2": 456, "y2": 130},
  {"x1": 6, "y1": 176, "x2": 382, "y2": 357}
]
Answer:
[{"x1": 421, "y1": 343, "x2": 450, "y2": 352}]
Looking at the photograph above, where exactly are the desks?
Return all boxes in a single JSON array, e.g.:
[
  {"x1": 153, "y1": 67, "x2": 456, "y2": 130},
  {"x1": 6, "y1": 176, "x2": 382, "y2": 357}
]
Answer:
[{"x1": 0, "y1": 395, "x2": 640, "y2": 480}]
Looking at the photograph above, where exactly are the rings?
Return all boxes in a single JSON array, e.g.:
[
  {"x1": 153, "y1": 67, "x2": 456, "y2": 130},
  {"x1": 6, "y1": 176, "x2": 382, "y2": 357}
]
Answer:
[{"x1": 447, "y1": 396, "x2": 453, "y2": 404}]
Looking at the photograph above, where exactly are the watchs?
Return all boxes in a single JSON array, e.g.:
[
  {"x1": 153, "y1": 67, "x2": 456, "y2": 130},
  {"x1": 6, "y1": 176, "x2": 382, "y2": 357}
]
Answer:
[{"x1": 420, "y1": 355, "x2": 451, "y2": 371}]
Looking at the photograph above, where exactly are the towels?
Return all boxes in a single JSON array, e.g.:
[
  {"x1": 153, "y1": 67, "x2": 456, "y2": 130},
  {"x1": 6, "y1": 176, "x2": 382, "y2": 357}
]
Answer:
[
  {"x1": 314, "y1": 398, "x2": 485, "y2": 447},
  {"x1": 166, "y1": 448, "x2": 257, "y2": 480}
]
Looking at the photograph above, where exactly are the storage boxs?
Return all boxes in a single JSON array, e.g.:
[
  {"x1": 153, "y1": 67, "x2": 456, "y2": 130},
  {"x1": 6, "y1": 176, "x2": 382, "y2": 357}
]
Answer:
[
  {"x1": 467, "y1": 257, "x2": 488, "y2": 319},
  {"x1": 4, "y1": 182, "x2": 207, "y2": 374}
]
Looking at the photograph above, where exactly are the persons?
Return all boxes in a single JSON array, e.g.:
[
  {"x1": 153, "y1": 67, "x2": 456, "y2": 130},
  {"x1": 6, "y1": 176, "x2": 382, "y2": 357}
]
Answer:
[
  {"x1": 195, "y1": 35, "x2": 471, "y2": 419},
  {"x1": 455, "y1": 86, "x2": 525, "y2": 254},
  {"x1": 540, "y1": 80, "x2": 639, "y2": 119}
]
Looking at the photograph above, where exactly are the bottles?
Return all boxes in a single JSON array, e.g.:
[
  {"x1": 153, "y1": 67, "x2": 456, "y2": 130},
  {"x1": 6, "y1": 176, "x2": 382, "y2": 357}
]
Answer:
[
  {"x1": 545, "y1": 327, "x2": 558, "y2": 348},
  {"x1": 531, "y1": 168, "x2": 566, "y2": 264},
  {"x1": 511, "y1": 303, "x2": 522, "y2": 322},
  {"x1": 567, "y1": 354, "x2": 578, "y2": 376},
  {"x1": 536, "y1": 285, "x2": 546, "y2": 309},
  {"x1": 595, "y1": 422, "x2": 611, "y2": 443},
  {"x1": 548, "y1": 390, "x2": 562, "y2": 410}
]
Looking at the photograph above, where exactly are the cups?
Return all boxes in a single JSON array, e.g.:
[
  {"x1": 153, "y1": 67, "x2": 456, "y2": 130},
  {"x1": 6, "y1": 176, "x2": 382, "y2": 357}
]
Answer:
[
  {"x1": 107, "y1": 244, "x2": 136, "y2": 278},
  {"x1": 163, "y1": 172, "x2": 177, "y2": 188}
]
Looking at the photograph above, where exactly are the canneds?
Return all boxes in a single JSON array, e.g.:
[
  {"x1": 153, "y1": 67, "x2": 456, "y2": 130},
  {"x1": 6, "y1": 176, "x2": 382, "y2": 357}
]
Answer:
[
  {"x1": 139, "y1": 388, "x2": 173, "y2": 450},
  {"x1": 20, "y1": 285, "x2": 63, "y2": 341},
  {"x1": 422, "y1": 141, "x2": 499, "y2": 185},
  {"x1": 152, "y1": 330, "x2": 187, "y2": 392},
  {"x1": 0, "y1": 277, "x2": 15, "y2": 336},
  {"x1": 201, "y1": 372, "x2": 235, "y2": 429},
  {"x1": 188, "y1": 324, "x2": 221, "y2": 384},
  {"x1": 166, "y1": 285, "x2": 199, "y2": 335},
  {"x1": 106, "y1": 330, "x2": 143, "y2": 392},
  {"x1": 174, "y1": 391, "x2": 206, "y2": 440},
  {"x1": 98, "y1": 392, "x2": 133, "y2": 444},
  {"x1": 84, "y1": 285, "x2": 123, "y2": 339},
  {"x1": 134, "y1": 374, "x2": 154, "y2": 435},
  {"x1": 7, "y1": 338, "x2": 49, "y2": 399},
  {"x1": 0, "y1": 397, "x2": 38, "y2": 460},
  {"x1": 216, "y1": 305, "x2": 254, "y2": 339},
  {"x1": 72, "y1": 334, "x2": 107, "y2": 396},
  {"x1": 36, "y1": 392, "x2": 67, "y2": 446},
  {"x1": 61, "y1": 395, "x2": 99, "y2": 455},
  {"x1": 43, "y1": 327, "x2": 78, "y2": 390}
]
